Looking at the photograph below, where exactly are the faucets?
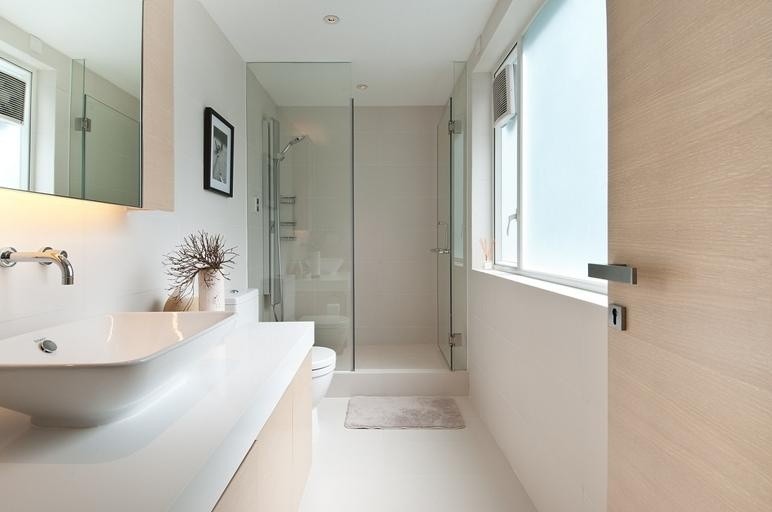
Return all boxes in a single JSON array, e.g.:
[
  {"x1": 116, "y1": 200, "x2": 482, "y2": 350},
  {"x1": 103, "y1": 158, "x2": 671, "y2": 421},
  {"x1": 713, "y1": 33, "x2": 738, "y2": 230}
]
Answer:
[{"x1": 0, "y1": 246, "x2": 74, "y2": 285}]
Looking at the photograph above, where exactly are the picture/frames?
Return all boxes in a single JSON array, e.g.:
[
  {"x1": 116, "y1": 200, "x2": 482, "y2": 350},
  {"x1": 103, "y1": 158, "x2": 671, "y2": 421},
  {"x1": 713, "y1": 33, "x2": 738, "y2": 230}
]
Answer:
[{"x1": 204, "y1": 107, "x2": 234, "y2": 198}]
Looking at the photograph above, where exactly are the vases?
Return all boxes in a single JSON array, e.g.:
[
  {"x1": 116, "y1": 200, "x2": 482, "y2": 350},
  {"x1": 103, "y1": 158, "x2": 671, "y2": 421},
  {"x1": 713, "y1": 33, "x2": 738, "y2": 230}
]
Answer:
[{"x1": 199, "y1": 268, "x2": 225, "y2": 311}]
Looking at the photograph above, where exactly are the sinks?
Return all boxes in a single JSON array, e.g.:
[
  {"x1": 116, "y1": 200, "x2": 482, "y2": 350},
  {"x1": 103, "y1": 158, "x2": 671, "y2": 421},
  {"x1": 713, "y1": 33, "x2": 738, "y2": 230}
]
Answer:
[
  {"x1": 0, "y1": 309, "x2": 242, "y2": 428},
  {"x1": 319, "y1": 256, "x2": 344, "y2": 273}
]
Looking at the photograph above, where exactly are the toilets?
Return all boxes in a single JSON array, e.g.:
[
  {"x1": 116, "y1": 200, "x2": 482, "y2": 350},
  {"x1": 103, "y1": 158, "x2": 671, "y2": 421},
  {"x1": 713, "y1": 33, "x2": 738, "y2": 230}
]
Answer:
[
  {"x1": 223, "y1": 288, "x2": 339, "y2": 446},
  {"x1": 274, "y1": 273, "x2": 350, "y2": 353}
]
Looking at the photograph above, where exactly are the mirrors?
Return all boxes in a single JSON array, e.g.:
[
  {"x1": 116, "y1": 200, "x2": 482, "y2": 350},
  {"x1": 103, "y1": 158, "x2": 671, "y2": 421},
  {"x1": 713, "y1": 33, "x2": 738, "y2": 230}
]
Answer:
[{"x1": 0, "y1": 0, "x2": 144, "y2": 208}]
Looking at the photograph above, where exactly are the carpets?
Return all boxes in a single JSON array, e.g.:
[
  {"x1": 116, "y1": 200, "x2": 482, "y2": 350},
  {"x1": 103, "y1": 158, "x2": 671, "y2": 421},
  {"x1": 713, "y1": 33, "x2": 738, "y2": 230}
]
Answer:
[{"x1": 344, "y1": 396, "x2": 465, "y2": 430}]
